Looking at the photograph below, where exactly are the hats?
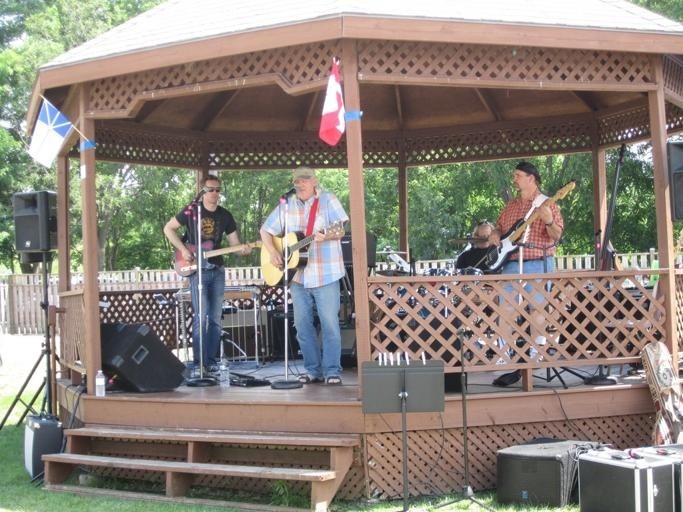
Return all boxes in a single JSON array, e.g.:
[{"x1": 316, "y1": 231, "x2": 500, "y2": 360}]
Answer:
[
  {"x1": 292, "y1": 166, "x2": 314, "y2": 183},
  {"x1": 516, "y1": 163, "x2": 540, "y2": 180}
]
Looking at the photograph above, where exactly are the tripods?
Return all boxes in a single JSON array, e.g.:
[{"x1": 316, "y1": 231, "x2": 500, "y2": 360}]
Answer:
[
  {"x1": 507, "y1": 243, "x2": 586, "y2": 390},
  {"x1": 1, "y1": 253, "x2": 61, "y2": 429},
  {"x1": 16, "y1": 373, "x2": 51, "y2": 425},
  {"x1": 424, "y1": 328, "x2": 499, "y2": 512}
]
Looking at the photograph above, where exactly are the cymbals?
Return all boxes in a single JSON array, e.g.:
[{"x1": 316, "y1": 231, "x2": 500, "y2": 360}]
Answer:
[{"x1": 449, "y1": 235, "x2": 490, "y2": 244}]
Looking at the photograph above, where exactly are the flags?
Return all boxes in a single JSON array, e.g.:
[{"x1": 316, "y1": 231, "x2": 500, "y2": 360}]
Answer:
[
  {"x1": 318, "y1": 61, "x2": 346, "y2": 146},
  {"x1": 27, "y1": 98, "x2": 74, "y2": 169}
]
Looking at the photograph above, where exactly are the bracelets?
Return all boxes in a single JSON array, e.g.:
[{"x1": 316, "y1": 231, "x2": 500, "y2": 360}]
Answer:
[{"x1": 544, "y1": 218, "x2": 555, "y2": 227}]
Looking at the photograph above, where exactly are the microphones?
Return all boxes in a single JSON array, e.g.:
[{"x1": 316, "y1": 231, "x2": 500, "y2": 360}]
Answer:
[
  {"x1": 192, "y1": 185, "x2": 210, "y2": 205},
  {"x1": 596, "y1": 229, "x2": 602, "y2": 235},
  {"x1": 618, "y1": 143, "x2": 628, "y2": 164},
  {"x1": 282, "y1": 187, "x2": 296, "y2": 199}
]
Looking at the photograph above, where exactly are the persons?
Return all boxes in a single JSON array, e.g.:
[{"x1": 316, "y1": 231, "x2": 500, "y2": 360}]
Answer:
[
  {"x1": 260, "y1": 166, "x2": 349, "y2": 385},
  {"x1": 491, "y1": 162, "x2": 565, "y2": 368},
  {"x1": 452, "y1": 217, "x2": 498, "y2": 273},
  {"x1": 162, "y1": 174, "x2": 253, "y2": 379}
]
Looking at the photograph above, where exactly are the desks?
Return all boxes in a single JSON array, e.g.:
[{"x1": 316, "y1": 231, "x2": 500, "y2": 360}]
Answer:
[{"x1": 177, "y1": 285, "x2": 264, "y2": 368}]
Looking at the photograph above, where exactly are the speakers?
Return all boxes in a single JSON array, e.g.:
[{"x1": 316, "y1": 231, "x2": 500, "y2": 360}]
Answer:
[
  {"x1": 666, "y1": 140, "x2": 683, "y2": 222},
  {"x1": 23, "y1": 408, "x2": 62, "y2": 480},
  {"x1": 496, "y1": 436, "x2": 597, "y2": 506},
  {"x1": 12, "y1": 191, "x2": 59, "y2": 254},
  {"x1": 342, "y1": 230, "x2": 377, "y2": 265},
  {"x1": 99, "y1": 321, "x2": 187, "y2": 393}
]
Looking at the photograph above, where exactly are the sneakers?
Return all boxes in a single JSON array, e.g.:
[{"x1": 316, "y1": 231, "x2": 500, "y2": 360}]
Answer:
[
  {"x1": 191, "y1": 364, "x2": 206, "y2": 378},
  {"x1": 207, "y1": 365, "x2": 221, "y2": 377}
]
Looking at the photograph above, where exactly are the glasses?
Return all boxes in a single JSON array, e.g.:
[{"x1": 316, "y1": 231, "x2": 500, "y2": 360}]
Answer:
[{"x1": 204, "y1": 185, "x2": 220, "y2": 192}]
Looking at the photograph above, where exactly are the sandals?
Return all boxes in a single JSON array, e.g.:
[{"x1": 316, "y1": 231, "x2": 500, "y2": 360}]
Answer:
[
  {"x1": 327, "y1": 376, "x2": 341, "y2": 385},
  {"x1": 299, "y1": 374, "x2": 323, "y2": 384}
]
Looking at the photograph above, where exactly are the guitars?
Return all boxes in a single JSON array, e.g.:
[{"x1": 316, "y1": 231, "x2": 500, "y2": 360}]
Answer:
[
  {"x1": 485, "y1": 180, "x2": 576, "y2": 272},
  {"x1": 260, "y1": 221, "x2": 344, "y2": 287},
  {"x1": 173, "y1": 241, "x2": 262, "y2": 276}
]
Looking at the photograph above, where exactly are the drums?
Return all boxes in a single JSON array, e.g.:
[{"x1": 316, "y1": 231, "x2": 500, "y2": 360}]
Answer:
[
  {"x1": 379, "y1": 302, "x2": 430, "y2": 355},
  {"x1": 373, "y1": 269, "x2": 417, "y2": 299},
  {"x1": 456, "y1": 267, "x2": 485, "y2": 299},
  {"x1": 423, "y1": 267, "x2": 456, "y2": 307}
]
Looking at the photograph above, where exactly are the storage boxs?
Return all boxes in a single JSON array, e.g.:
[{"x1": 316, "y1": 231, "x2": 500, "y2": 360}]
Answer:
[{"x1": 576, "y1": 447, "x2": 676, "y2": 512}]
